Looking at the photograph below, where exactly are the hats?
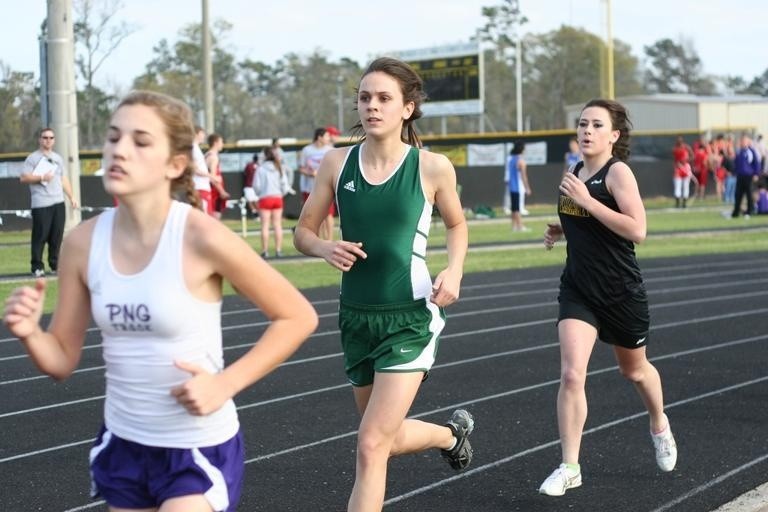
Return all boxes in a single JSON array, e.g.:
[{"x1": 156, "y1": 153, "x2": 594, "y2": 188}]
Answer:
[{"x1": 326, "y1": 127, "x2": 341, "y2": 135}]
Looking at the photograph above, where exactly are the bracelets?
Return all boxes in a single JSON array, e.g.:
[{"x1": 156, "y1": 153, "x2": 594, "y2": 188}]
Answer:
[{"x1": 40, "y1": 176, "x2": 44, "y2": 181}]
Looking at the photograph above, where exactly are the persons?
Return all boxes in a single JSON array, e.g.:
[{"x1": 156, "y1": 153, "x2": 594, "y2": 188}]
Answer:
[
  {"x1": 2, "y1": 90, "x2": 320, "y2": 512},
  {"x1": 540, "y1": 99, "x2": 679, "y2": 498},
  {"x1": 186, "y1": 128, "x2": 342, "y2": 258},
  {"x1": 294, "y1": 57, "x2": 474, "y2": 512},
  {"x1": 671, "y1": 133, "x2": 768, "y2": 219},
  {"x1": 505, "y1": 143, "x2": 531, "y2": 231},
  {"x1": 20, "y1": 128, "x2": 77, "y2": 278},
  {"x1": 564, "y1": 140, "x2": 581, "y2": 171}
]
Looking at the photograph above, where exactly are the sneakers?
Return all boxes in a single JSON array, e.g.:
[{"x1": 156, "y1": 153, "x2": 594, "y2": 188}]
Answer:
[
  {"x1": 650, "y1": 413, "x2": 678, "y2": 472},
  {"x1": 539, "y1": 463, "x2": 582, "y2": 496},
  {"x1": 31, "y1": 268, "x2": 56, "y2": 277},
  {"x1": 441, "y1": 409, "x2": 475, "y2": 470},
  {"x1": 260, "y1": 251, "x2": 282, "y2": 259}
]
